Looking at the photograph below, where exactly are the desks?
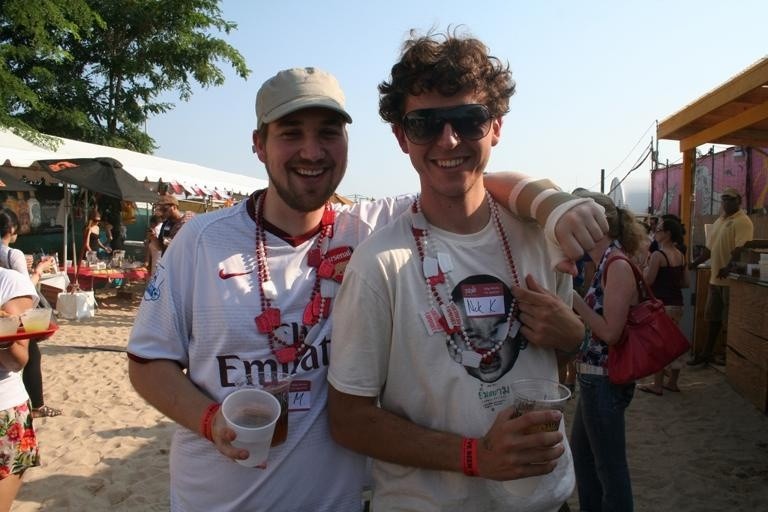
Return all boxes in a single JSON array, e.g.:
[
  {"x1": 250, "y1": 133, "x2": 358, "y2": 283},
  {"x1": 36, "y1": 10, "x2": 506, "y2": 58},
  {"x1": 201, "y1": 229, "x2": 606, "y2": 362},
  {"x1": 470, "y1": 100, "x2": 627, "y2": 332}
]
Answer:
[{"x1": 60, "y1": 264, "x2": 153, "y2": 295}]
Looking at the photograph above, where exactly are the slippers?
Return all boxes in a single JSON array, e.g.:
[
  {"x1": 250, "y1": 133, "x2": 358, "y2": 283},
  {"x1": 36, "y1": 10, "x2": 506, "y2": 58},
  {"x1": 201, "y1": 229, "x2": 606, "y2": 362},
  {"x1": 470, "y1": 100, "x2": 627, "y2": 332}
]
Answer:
[
  {"x1": 636, "y1": 383, "x2": 663, "y2": 396},
  {"x1": 662, "y1": 382, "x2": 680, "y2": 392}
]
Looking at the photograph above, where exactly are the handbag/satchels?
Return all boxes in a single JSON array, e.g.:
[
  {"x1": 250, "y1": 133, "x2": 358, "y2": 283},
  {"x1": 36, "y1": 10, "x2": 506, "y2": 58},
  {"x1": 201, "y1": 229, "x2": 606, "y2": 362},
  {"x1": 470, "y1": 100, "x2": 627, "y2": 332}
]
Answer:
[
  {"x1": 8, "y1": 248, "x2": 58, "y2": 342},
  {"x1": 603, "y1": 255, "x2": 691, "y2": 385}
]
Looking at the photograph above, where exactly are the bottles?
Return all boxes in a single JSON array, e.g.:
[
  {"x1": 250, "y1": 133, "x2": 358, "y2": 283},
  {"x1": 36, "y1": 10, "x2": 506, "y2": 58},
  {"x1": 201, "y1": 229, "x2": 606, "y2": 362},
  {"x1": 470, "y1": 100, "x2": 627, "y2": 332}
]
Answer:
[{"x1": 118, "y1": 254, "x2": 135, "y2": 267}]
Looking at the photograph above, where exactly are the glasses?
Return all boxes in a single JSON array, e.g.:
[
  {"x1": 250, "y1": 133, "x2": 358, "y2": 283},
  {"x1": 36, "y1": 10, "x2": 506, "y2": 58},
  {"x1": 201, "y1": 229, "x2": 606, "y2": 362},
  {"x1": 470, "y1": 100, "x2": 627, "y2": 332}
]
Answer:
[
  {"x1": 402, "y1": 103, "x2": 495, "y2": 145},
  {"x1": 655, "y1": 227, "x2": 664, "y2": 233},
  {"x1": 90, "y1": 218, "x2": 101, "y2": 223}
]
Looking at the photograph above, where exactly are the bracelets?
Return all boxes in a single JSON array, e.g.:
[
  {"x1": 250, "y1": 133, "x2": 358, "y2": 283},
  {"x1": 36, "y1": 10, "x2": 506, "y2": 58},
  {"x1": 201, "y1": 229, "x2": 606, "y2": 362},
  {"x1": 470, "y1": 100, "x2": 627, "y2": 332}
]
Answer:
[
  {"x1": 1, "y1": 345, "x2": 11, "y2": 351},
  {"x1": 199, "y1": 400, "x2": 219, "y2": 444},
  {"x1": 457, "y1": 433, "x2": 481, "y2": 476}
]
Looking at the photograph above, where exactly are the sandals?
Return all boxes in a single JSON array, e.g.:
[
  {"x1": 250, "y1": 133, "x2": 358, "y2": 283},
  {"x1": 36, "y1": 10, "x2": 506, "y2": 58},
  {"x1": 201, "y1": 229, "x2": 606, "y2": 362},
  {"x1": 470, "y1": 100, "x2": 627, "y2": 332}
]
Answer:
[{"x1": 32, "y1": 406, "x2": 62, "y2": 418}]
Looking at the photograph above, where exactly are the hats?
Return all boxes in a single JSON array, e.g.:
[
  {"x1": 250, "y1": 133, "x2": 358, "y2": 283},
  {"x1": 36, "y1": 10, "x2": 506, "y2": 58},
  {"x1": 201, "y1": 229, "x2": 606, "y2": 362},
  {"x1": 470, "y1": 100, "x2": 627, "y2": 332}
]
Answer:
[
  {"x1": 256, "y1": 67, "x2": 352, "y2": 133},
  {"x1": 153, "y1": 195, "x2": 179, "y2": 206},
  {"x1": 721, "y1": 188, "x2": 742, "y2": 199}
]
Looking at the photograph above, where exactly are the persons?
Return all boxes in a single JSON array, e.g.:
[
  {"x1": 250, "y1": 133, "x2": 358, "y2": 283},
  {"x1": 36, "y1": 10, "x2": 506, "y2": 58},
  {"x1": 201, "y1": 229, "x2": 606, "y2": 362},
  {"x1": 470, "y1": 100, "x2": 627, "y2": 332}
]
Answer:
[
  {"x1": 82, "y1": 209, "x2": 164, "y2": 276},
  {"x1": 157, "y1": 193, "x2": 195, "y2": 254},
  {"x1": 323, "y1": 25, "x2": 586, "y2": 512},
  {"x1": 123, "y1": 63, "x2": 609, "y2": 509},
  {"x1": 686, "y1": 187, "x2": 757, "y2": 374},
  {"x1": 0, "y1": 206, "x2": 64, "y2": 420},
  {"x1": 569, "y1": 187, "x2": 689, "y2": 397},
  {"x1": 567, "y1": 185, "x2": 641, "y2": 510},
  {"x1": 0, "y1": 265, "x2": 43, "y2": 512}
]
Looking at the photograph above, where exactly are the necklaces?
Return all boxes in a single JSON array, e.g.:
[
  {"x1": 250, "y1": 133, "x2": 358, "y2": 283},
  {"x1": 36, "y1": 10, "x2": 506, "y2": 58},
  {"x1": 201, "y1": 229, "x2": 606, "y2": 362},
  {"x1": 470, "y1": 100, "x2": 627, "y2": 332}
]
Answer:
[
  {"x1": 399, "y1": 187, "x2": 528, "y2": 374},
  {"x1": 250, "y1": 188, "x2": 337, "y2": 372}
]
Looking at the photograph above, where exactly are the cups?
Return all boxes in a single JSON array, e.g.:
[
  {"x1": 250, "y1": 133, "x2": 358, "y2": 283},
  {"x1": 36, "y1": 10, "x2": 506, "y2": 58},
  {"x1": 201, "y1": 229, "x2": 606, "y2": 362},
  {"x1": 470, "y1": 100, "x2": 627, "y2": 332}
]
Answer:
[
  {"x1": 0, "y1": 305, "x2": 52, "y2": 336},
  {"x1": 237, "y1": 373, "x2": 292, "y2": 447},
  {"x1": 503, "y1": 379, "x2": 570, "y2": 499},
  {"x1": 223, "y1": 391, "x2": 278, "y2": 466},
  {"x1": 41, "y1": 257, "x2": 50, "y2": 274}
]
